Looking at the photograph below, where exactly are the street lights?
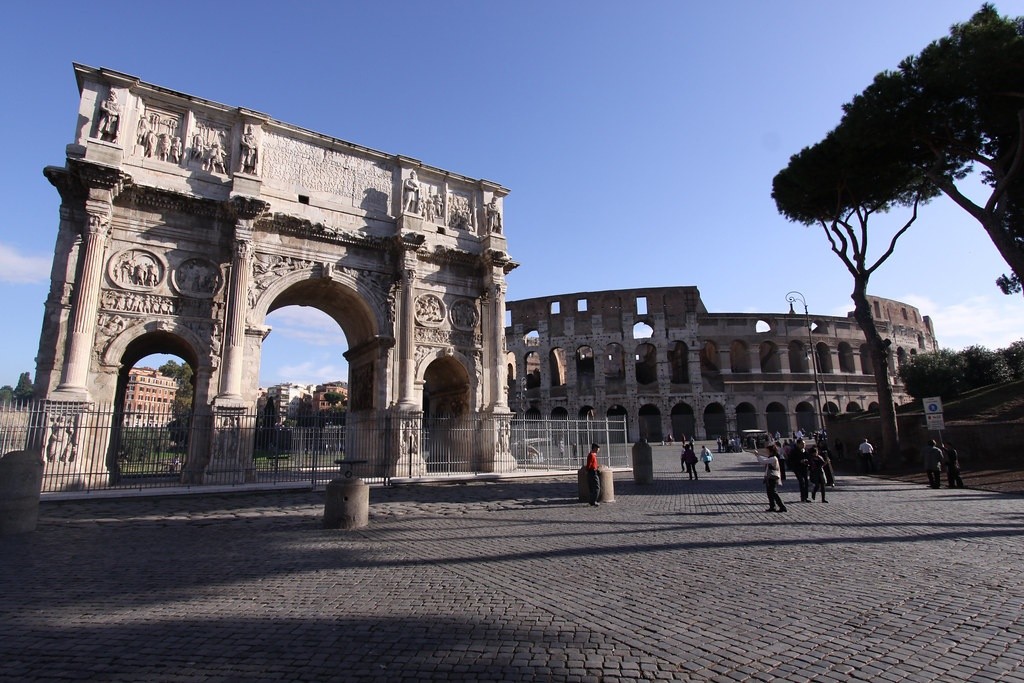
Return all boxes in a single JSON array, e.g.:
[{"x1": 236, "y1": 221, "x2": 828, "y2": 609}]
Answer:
[
  {"x1": 785, "y1": 291, "x2": 828, "y2": 445},
  {"x1": 804, "y1": 340, "x2": 829, "y2": 413}
]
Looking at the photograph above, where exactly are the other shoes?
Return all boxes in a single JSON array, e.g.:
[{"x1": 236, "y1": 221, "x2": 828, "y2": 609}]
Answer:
[
  {"x1": 825, "y1": 483, "x2": 831, "y2": 486},
  {"x1": 590, "y1": 502, "x2": 600, "y2": 506},
  {"x1": 831, "y1": 483, "x2": 835, "y2": 487},
  {"x1": 801, "y1": 498, "x2": 811, "y2": 503},
  {"x1": 777, "y1": 510, "x2": 788, "y2": 512},
  {"x1": 766, "y1": 509, "x2": 777, "y2": 512},
  {"x1": 822, "y1": 500, "x2": 829, "y2": 503},
  {"x1": 812, "y1": 490, "x2": 816, "y2": 500}
]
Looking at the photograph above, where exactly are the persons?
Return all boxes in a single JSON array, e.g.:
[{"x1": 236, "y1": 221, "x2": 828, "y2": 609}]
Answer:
[
  {"x1": 586, "y1": 444, "x2": 601, "y2": 507},
  {"x1": 172, "y1": 427, "x2": 963, "y2": 513}
]
